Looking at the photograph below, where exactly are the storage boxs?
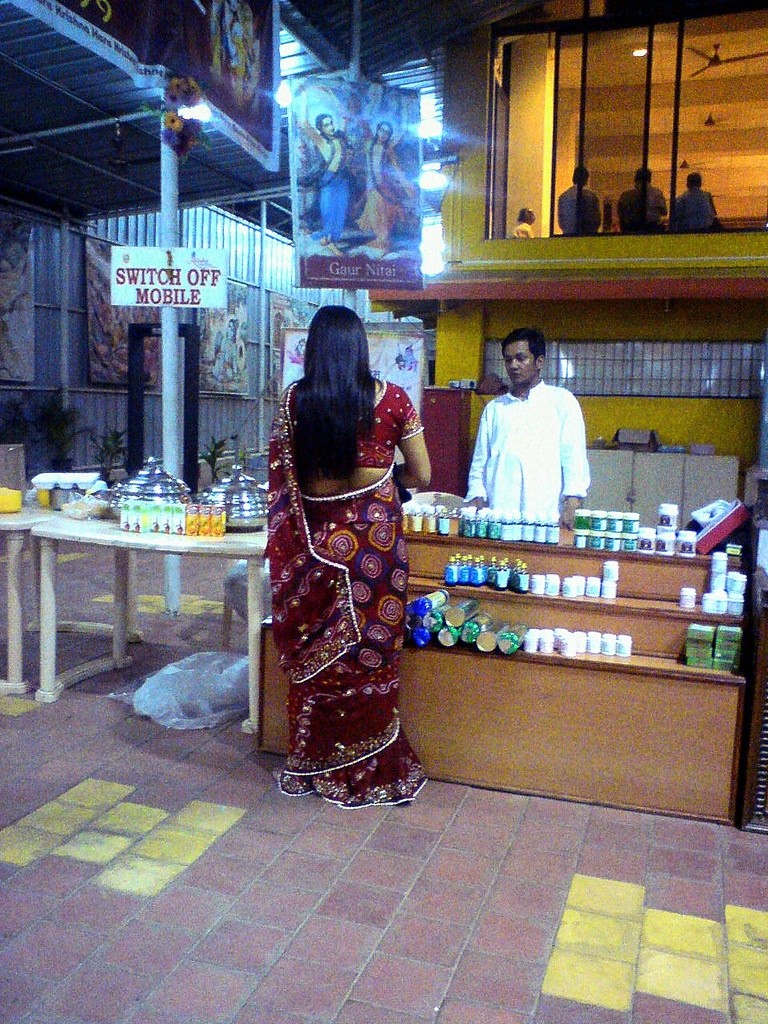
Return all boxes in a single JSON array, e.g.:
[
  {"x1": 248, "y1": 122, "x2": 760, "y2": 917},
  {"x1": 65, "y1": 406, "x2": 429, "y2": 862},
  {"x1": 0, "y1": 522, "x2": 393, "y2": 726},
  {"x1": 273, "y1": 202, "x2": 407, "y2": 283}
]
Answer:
[
  {"x1": 696, "y1": 498, "x2": 751, "y2": 555},
  {"x1": 611, "y1": 426, "x2": 662, "y2": 453},
  {"x1": 0, "y1": 445, "x2": 25, "y2": 504}
]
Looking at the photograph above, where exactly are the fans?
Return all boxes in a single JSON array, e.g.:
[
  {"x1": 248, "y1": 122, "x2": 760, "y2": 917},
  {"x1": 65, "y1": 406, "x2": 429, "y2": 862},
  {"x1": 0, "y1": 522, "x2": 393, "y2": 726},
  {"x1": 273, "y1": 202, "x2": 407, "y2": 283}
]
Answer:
[
  {"x1": 683, "y1": 44, "x2": 768, "y2": 78},
  {"x1": 651, "y1": 111, "x2": 724, "y2": 172}
]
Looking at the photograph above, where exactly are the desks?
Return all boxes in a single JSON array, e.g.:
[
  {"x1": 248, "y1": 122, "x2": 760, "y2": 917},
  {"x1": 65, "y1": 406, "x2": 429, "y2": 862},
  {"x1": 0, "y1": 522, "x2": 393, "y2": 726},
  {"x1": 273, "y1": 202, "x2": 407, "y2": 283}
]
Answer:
[{"x1": 0, "y1": 504, "x2": 271, "y2": 734}]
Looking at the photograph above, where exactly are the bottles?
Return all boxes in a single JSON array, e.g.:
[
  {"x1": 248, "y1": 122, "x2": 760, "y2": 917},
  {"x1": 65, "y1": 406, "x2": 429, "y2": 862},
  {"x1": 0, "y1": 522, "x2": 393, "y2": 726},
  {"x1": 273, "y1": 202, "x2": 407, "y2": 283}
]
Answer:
[
  {"x1": 443, "y1": 553, "x2": 529, "y2": 593},
  {"x1": 679, "y1": 551, "x2": 747, "y2": 615},
  {"x1": 573, "y1": 503, "x2": 697, "y2": 558},
  {"x1": 524, "y1": 628, "x2": 633, "y2": 657},
  {"x1": 531, "y1": 560, "x2": 620, "y2": 599},
  {"x1": 402, "y1": 504, "x2": 560, "y2": 544}
]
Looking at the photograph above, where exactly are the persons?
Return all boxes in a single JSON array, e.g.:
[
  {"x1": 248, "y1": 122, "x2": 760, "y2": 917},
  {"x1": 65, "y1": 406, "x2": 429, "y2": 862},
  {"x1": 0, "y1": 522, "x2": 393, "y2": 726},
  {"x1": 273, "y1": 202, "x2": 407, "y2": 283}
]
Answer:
[
  {"x1": 512, "y1": 208, "x2": 535, "y2": 238},
  {"x1": 264, "y1": 307, "x2": 432, "y2": 809},
  {"x1": 674, "y1": 172, "x2": 717, "y2": 233},
  {"x1": 617, "y1": 167, "x2": 668, "y2": 233},
  {"x1": 558, "y1": 167, "x2": 601, "y2": 235},
  {"x1": 463, "y1": 326, "x2": 591, "y2": 529}
]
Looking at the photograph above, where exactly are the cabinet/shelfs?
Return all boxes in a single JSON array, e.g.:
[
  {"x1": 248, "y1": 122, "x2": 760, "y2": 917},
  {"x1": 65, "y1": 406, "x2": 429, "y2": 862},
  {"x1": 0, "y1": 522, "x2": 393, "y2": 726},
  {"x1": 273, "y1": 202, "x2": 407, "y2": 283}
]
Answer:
[
  {"x1": 257, "y1": 519, "x2": 746, "y2": 826},
  {"x1": 579, "y1": 451, "x2": 738, "y2": 532}
]
